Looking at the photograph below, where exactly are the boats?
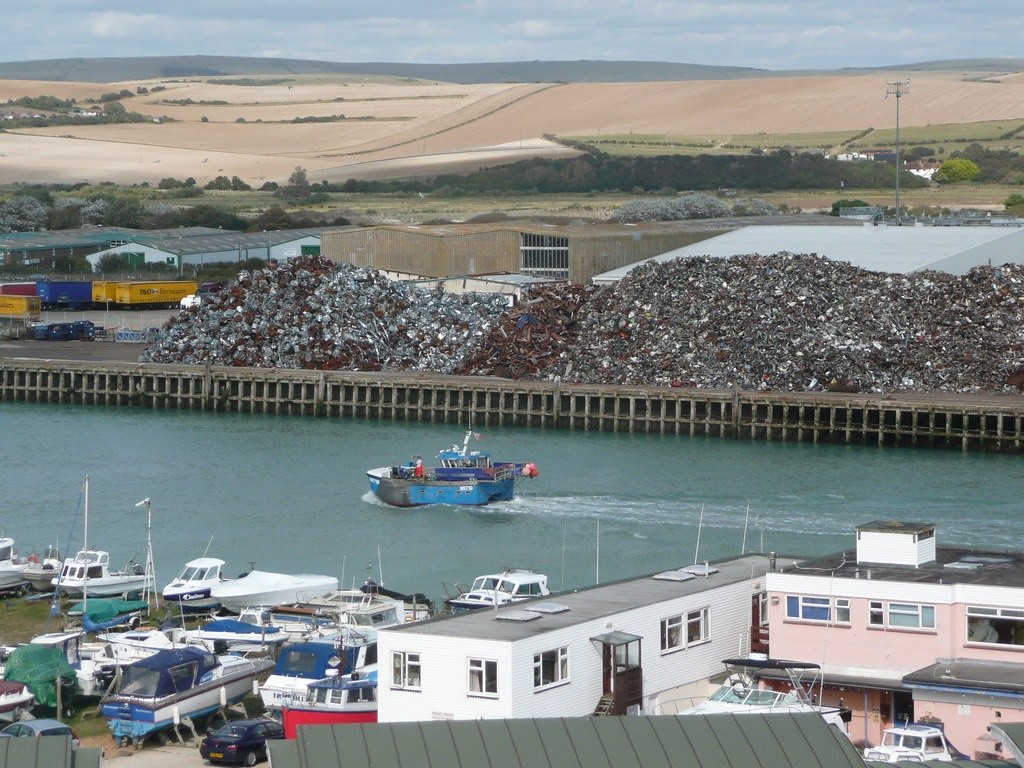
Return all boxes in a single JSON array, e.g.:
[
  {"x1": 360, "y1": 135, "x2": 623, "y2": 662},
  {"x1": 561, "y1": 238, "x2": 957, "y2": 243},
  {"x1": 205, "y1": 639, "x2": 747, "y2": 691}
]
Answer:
[
  {"x1": 366, "y1": 399, "x2": 539, "y2": 508},
  {"x1": 861, "y1": 717, "x2": 971, "y2": 764},
  {"x1": 21, "y1": 534, "x2": 71, "y2": 594},
  {"x1": 648, "y1": 634, "x2": 853, "y2": 741},
  {"x1": 50, "y1": 546, "x2": 154, "y2": 601},
  {"x1": 0, "y1": 473, "x2": 438, "y2": 751},
  {"x1": 210, "y1": 570, "x2": 339, "y2": 617},
  {"x1": 0, "y1": 521, "x2": 36, "y2": 597},
  {"x1": 162, "y1": 533, "x2": 255, "y2": 602},
  {"x1": 441, "y1": 566, "x2": 558, "y2": 616}
]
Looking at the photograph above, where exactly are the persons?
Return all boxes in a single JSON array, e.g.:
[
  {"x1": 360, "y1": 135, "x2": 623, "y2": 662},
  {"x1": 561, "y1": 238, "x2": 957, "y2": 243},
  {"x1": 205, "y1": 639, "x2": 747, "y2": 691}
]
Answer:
[{"x1": 416, "y1": 456, "x2": 422, "y2": 478}]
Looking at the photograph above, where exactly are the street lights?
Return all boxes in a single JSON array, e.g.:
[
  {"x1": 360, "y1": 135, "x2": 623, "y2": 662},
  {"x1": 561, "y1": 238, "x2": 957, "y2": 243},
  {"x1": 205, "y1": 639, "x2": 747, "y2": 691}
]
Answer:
[{"x1": 106, "y1": 298, "x2": 113, "y2": 328}]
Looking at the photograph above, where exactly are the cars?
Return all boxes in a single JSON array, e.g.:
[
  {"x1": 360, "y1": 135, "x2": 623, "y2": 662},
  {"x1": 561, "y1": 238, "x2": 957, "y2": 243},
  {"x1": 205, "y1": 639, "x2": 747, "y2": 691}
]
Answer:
[
  {"x1": 1, "y1": 718, "x2": 80, "y2": 750},
  {"x1": 200, "y1": 719, "x2": 285, "y2": 768}
]
